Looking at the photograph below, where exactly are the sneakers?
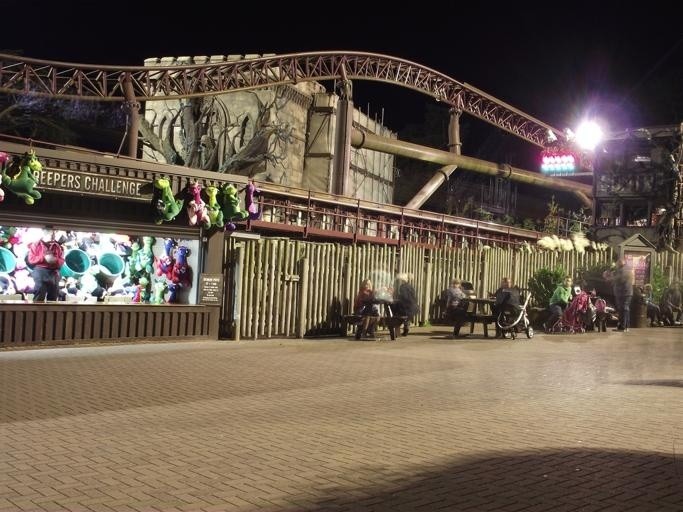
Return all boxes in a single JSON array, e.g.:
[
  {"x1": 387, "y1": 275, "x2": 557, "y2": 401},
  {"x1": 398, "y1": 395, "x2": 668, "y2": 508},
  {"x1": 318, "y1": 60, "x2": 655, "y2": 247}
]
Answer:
[
  {"x1": 612, "y1": 327, "x2": 628, "y2": 332},
  {"x1": 652, "y1": 321, "x2": 681, "y2": 326}
]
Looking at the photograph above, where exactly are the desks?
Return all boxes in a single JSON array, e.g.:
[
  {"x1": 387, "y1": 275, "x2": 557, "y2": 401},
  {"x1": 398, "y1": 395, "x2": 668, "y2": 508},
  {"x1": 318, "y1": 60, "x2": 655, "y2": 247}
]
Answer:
[
  {"x1": 355, "y1": 300, "x2": 396, "y2": 340},
  {"x1": 453, "y1": 298, "x2": 496, "y2": 337}
]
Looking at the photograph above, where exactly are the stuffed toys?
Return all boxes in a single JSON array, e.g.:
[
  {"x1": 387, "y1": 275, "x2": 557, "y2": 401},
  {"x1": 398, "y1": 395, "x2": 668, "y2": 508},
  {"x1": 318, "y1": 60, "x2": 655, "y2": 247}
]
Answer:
[
  {"x1": 0, "y1": 225, "x2": 192, "y2": 304},
  {"x1": 222, "y1": 182, "x2": 250, "y2": 230},
  {"x1": 2, "y1": 149, "x2": 44, "y2": 206},
  {"x1": 152, "y1": 177, "x2": 184, "y2": 225},
  {"x1": 203, "y1": 185, "x2": 224, "y2": 230},
  {"x1": 247, "y1": 181, "x2": 263, "y2": 220},
  {"x1": 187, "y1": 180, "x2": 211, "y2": 226}
]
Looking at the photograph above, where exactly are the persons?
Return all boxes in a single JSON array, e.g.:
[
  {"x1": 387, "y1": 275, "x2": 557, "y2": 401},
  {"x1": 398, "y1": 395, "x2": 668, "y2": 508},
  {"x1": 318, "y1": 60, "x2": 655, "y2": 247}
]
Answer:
[
  {"x1": 449, "y1": 280, "x2": 478, "y2": 337},
  {"x1": 446, "y1": 278, "x2": 466, "y2": 311},
  {"x1": 353, "y1": 278, "x2": 379, "y2": 338},
  {"x1": 543, "y1": 275, "x2": 574, "y2": 331},
  {"x1": 388, "y1": 273, "x2": 420, "y2": 337},
  {"x1": 489, "y1": 277, "x2": 513, "y2": 338},
  {"x1": 637, "y1": 281, "x2": 683, "y2": 326},
  {"x1": 603, "y1": 258, "x2": 634, "y2": 333}
]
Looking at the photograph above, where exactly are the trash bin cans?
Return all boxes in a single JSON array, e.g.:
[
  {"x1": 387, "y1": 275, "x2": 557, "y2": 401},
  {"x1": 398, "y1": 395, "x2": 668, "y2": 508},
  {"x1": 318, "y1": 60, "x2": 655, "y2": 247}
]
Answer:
[{"x1": 218, "y1": 319, "x2": 235, "y2": 339}]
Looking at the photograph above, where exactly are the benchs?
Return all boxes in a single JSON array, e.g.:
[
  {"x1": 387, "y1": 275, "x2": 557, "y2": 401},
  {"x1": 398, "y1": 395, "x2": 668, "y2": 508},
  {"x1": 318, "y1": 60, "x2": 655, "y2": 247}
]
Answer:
[
  {"x1": 344, "y1": 315, "x2": 408, "y2": 340},
  {"x1": 441, "y1": 311, "x2": 498, "y2": 337}
]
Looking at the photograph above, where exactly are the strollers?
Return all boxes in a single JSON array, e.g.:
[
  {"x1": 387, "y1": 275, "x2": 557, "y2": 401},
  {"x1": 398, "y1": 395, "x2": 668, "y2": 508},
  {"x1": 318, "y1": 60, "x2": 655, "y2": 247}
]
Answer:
[{"x1": 495, "y1": 285, "x2": 534, "y2": 338}]
